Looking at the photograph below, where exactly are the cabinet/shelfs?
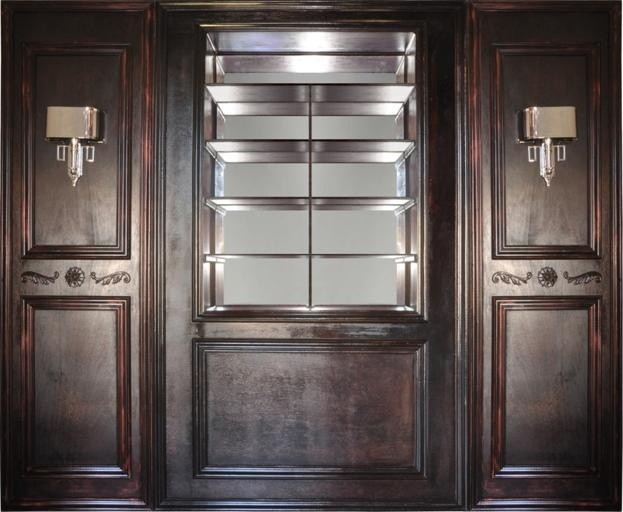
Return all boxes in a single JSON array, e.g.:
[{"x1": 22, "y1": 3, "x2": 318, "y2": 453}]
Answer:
[{"x1": 198, "y1": 25, "x2": 421, "y2": 314}]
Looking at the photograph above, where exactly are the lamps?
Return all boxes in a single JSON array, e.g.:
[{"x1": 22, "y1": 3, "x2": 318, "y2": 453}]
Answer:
[
  {"x1": 520, "y1": 105, "x2": 577, "y2": 189},
  {"x1": 46, "y1": 107, "x2": 102, "y2": 189}
]
